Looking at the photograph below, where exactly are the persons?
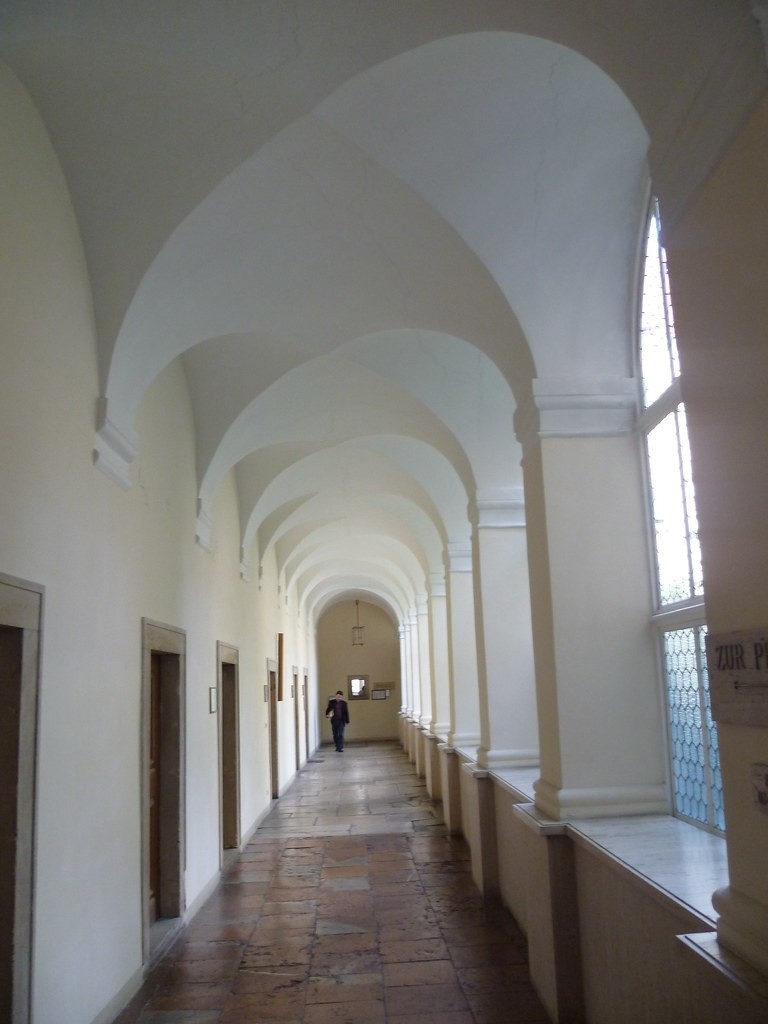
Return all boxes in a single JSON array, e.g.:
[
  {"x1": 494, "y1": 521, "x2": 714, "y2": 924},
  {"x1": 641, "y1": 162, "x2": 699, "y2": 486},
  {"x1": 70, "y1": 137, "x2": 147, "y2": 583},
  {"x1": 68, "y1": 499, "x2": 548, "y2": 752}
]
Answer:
[{"x1": 326, "y1": 690, "x2": 350, "y2": 752}]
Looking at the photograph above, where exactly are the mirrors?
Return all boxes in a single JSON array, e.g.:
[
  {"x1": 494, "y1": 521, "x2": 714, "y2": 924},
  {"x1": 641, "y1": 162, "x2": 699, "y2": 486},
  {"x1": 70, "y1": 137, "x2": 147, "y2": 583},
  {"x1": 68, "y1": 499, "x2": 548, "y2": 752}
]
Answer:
[{"x1": 347, "y1": 674, "x2": 369, "y2": 700}]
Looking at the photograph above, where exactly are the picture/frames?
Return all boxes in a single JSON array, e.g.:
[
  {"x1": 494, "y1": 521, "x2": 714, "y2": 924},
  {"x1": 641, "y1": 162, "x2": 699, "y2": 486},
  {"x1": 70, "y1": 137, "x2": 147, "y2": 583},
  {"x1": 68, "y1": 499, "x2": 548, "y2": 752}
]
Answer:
[
  {"x1": 209, "y1": 686, "x2": 217, "y2": 713},
  {"x1": 371, "y1": 689, "x2": 386, "y2": 700}
]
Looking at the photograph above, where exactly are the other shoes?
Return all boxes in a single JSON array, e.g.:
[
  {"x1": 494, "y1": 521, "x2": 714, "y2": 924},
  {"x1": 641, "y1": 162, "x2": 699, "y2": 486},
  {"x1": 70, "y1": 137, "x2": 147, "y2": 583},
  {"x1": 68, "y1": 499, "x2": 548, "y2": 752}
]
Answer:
[{"x1": 336, "y1": 748, "x2": 344, "y2": 752}]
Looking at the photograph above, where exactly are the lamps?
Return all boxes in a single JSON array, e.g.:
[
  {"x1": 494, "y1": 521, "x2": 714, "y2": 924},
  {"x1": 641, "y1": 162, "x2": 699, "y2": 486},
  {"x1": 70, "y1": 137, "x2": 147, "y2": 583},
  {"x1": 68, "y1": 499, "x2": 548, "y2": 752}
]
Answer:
[{"x1": 352, "y1": 600, "x2": 365, "y2": 646}]
